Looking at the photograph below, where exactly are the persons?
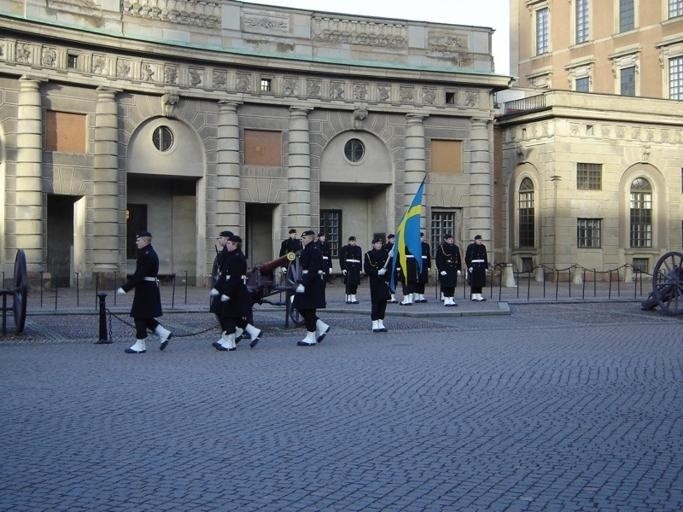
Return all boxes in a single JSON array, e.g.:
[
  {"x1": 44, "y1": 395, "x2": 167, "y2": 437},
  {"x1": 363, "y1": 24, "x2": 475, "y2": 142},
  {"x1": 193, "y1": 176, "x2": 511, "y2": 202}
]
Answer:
[
  {"x1": 209, "y1": 235, "x2": 264, "y2": 351},
  {"x1": 279, "y1": 227, "x2": 302, "y2": 275},
  {"x1": 209, "y1": 230, "x2": 247, "y2": 347},
  {"x1": 400, "y1": 246, "x2": 414, "y2": 305},
  {"x1": 338, "y1": 235, "x2": 363, "y2": 304},
  {"x1": 364, "y1": 236, "x2": 393, "y2": 332},
  {"x1": 115, "y1": 231, "x2": 173, "y2": 352},
  {"x1": 435, "y1": 233, "x2": 489, "y2": 307},
  {"x1": 293, "y1": 230, "x2": 331, "y2": 347},
  {"x1": 414, "y1": 232, "x2": 431, "y2": 303},
  {"x1": 386, "y1": 233, "x2": 401, "y2": 303},
  {"x1": 318, "y1": 232, "x2": 333, "y2": 307}
]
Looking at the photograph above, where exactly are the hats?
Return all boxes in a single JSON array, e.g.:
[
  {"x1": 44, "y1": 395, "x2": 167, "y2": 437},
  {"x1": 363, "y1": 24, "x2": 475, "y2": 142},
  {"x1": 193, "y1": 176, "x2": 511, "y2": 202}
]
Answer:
[
  {"x1": 136, "y1": 231, "x2": 152, "y2": 239},
  {"x1": 475, "y1": 235, "x2": 482, "y2": 240},
  {"x1": 372, "y1": 236, "x2": 384, "y2": 243},
  {"x1": 301, "y1": 230, "x2": 314, "y2": 236},
  {"x1": 387, "y1": 234, "x2": 395, "y2": 239},
  {"x1": 219, "y1": 230, "x2": 234, "y2": 238},
  {"x1": 318, "y1": 231, "x2": 326, "y2": 237},
  {"x1": 227, "y1": 234, "x2": 243, "y2": 243},
  {"x1": 349, "y1": 236, "x2": 356, "y2": 241},
  {"x1": 289, "y1": 229, "x2": 297, "y2": 234},
  {"x1": 443, "y1": 234, "x2": 451, "y2": 240}
]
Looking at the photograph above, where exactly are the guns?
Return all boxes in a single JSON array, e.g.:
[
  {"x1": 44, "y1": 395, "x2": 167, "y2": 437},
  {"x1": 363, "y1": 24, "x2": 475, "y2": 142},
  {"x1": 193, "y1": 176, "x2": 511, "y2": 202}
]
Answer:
[{"x1": 214, "y1": 244, "x2": 223, "y2": 274}]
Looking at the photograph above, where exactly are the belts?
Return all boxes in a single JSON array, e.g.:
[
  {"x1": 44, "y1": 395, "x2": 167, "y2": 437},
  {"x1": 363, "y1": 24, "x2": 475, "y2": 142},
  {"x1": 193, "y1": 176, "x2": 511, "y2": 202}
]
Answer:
[
  {"x1": 225, "y1": 273, "x2": 248, "y2": 285},
  {"x1": 471, "y1": 259, "x2": 485, "y2": 263},
  {"x1": 322, "y1": 256, "x2": 329, "y2": 259},
  {"x1": 346, "y1": 259, "x2": 361, "y2": 263},
  {"x1": 421, "y1": 256, "x2": 428, "y2": 259},
  {"x1": 145, "y1": 276, "x2": 160, "y2": 287},
  {"x1": 302, "y1": 269, "x2": 325, "y2": 280},
  {"x1": 405, "y1": 255, "x2": 415, "y2": 258}
]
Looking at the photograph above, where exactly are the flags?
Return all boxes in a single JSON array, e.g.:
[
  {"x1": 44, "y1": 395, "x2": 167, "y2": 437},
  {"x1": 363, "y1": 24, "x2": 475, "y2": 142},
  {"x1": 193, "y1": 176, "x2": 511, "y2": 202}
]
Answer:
[{"x1": 388, "y1": 179, "x2": 426, "y2": 292}]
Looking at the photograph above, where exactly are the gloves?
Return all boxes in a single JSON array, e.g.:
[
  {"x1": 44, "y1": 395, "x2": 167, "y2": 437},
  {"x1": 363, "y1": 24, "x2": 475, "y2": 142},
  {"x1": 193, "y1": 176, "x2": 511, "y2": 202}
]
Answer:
[
  {"x1": 296, "y1": 284, "x2": 305, "y2": 292},
  {"x1": 457, "y1": 270, "x2": 461, "y2": 275},
  {"x1": 469, "y1": 267, "x2": 473, "y2": 273},
  {"x1": 342, "y1": 269, "x2": 348, "y2": 276},
  {"x1": 220, "y1": 294, "x2": 230, "y2": 302},
  {"x1": 440, "y1": 271, "x2": 448, "y2": 276},
  {"x1": 117, "y1": 287, "x2": 127, "y2": 297},
  {"x1": 378, "y1": 267, "x2": 386, "y2": 275},
  {"x1": 210, "y1": 288, "x2": 220, "y2": 296}
]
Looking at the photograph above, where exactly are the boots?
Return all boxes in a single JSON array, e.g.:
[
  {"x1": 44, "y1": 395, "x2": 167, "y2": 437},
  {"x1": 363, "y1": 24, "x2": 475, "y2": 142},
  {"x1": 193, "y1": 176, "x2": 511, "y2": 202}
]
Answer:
[
  {"x1": 345, "y1": 294, "x2": 352, "y2": 304},
  {"x1": 378, "y1": 318, "x2": 389, "y2": 333},
  {"x1": 471, "y1": 294, "x2": 480, "y2": 301},
  {"x1": 444, "y1": 297, "x2": 451, "y2": 306},
  {"x1": 386, "y1": 291, "x2": 427, "y2": 305},
  {"x1": 315, "y1": 320, "x2": 331, "y2": 343},
  {"x1": 213, "y1": 324, "x2": 263, "y2": 352},
  {"x1": 154, "y1": 324, "x2": 173, "y2": 351},
  {"x1": 297, "y1": 330, "x2": 318, "y2": 346},
  {"x1": 450, "y1": 296, "x2": 455, "y2": 304},
  {"x1": 124, "y1": 338, "x2": 147, "y2": 353},
  {"x1": 478, "y1": 293, "x2": 485, "y2": 301},
  {"x1": 372, "y1": 319, "x2": 381, "y2": 333},
  {"x1": 351, "y1": 294, "x2": 359, "y2": 304}
]
